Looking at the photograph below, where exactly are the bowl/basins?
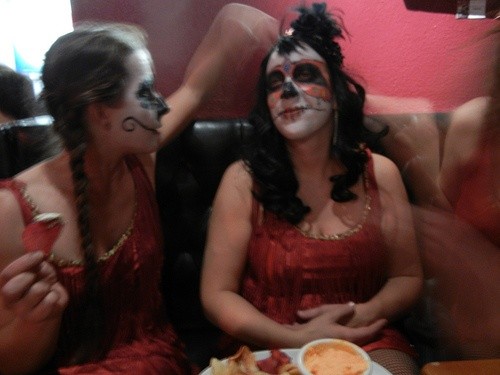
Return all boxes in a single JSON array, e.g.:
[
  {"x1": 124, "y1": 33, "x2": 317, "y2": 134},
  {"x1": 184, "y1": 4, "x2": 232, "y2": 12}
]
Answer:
[{"x1": 297, "y1": 339, "x2": 373, "y2": 375}]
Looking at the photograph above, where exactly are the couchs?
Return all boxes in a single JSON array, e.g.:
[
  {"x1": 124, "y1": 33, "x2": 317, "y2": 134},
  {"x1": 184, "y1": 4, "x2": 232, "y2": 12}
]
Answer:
[{"x1": 0, "y1": 110, "x2": 466, "y2": 366}]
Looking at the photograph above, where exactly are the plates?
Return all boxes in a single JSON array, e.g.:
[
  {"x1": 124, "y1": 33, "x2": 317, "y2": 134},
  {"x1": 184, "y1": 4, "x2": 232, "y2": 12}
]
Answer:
[{"x1": 199, "y1": 349, "x2": 392, "y2": 375}]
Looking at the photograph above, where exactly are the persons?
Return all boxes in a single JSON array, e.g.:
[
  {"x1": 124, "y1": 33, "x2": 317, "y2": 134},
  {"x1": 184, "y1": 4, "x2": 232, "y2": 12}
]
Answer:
[
  {"x1": 0, "y1": 4, "x2": 282, "y2": 375},
  {"x1": 200, "y1": 3, "x2": 424, "y2": 375},
  {"x1": 391, "y1": 16, "x2": 500, "y2": 359}
]
